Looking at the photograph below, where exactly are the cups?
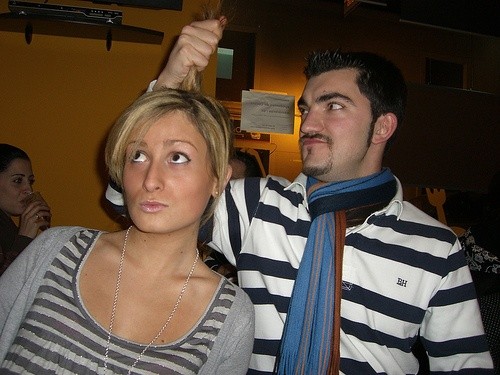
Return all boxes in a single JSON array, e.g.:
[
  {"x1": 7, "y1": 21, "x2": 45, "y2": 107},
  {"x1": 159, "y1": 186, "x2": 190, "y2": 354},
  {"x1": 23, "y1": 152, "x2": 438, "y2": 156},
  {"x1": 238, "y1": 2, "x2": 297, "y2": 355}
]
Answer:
[{"x1": 20, "y1": 191, "x2": 52, "y2": 231}]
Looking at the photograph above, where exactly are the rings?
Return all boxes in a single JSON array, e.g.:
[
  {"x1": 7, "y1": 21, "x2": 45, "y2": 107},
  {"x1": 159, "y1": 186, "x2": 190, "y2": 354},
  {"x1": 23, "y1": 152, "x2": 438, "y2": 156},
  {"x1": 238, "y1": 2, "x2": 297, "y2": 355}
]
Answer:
[{"x1": 35, "y1": 215, "x2": 39, "y2": 219}]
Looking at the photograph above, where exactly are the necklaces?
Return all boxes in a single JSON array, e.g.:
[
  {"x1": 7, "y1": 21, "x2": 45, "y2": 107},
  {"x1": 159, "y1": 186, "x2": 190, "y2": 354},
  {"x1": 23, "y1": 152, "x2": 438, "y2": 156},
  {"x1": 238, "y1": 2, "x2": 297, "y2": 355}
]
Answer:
[{"x1": 103, "y1": 226, "x2": 199, "y2": 375}]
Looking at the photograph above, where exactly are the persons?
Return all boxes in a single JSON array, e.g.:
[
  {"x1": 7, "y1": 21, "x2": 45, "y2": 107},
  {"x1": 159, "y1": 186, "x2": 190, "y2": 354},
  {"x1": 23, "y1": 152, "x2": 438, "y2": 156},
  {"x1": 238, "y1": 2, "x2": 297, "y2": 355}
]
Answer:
[
  {"x1": 0, "y1": 144, "x2": 52, "y2": 276},
  {"x1": 105, "y1": 19, "x2": 494, "y2": 375},
  {"x1": 0, "y1": 87, "x2": 255, "y2": 375},
  {"x1": 204, "y1": 151, "x2": 262, "y2": 284}
]
